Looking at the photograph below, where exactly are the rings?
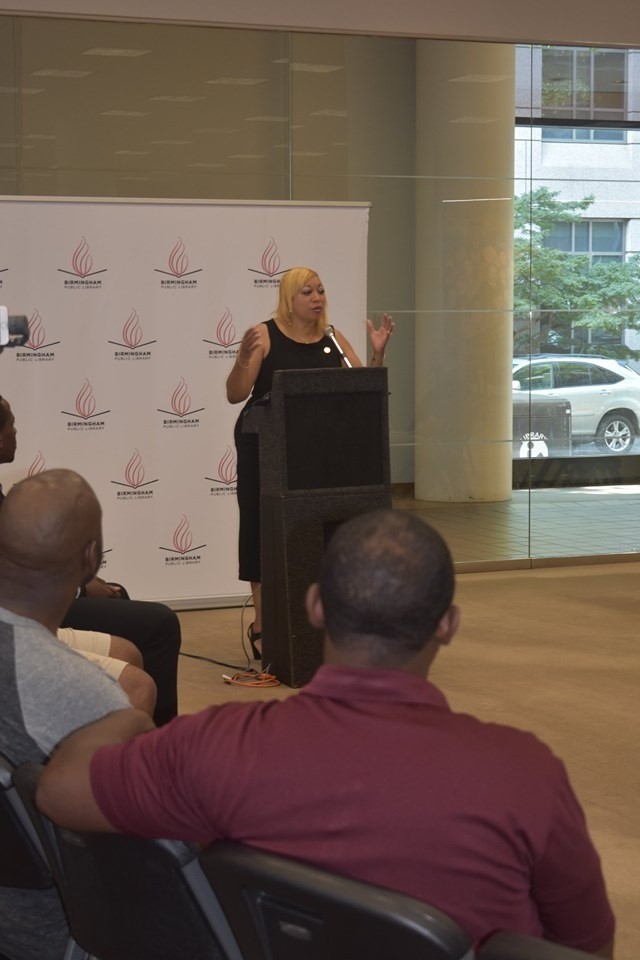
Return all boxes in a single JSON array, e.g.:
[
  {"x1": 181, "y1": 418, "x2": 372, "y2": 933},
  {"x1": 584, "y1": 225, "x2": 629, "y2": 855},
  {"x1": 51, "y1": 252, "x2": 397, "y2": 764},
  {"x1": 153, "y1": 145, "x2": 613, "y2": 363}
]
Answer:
[{"x1": 387, "y1": 331, "x2": 392, "y2": 335}]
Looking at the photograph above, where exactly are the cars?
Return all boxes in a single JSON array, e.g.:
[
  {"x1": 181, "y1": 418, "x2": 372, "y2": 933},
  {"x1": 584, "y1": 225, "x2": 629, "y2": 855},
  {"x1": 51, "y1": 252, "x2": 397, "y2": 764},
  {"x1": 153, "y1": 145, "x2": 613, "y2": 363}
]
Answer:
[{"x1": 512, "y1": 350, "x2": 639, "y2": 452}]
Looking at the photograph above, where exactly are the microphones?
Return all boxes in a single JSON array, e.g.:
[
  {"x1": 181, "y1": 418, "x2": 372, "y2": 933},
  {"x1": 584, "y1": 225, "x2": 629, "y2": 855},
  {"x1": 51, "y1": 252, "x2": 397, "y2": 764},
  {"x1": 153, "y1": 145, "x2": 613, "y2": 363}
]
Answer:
[{"x1": 323, "y1": 326, "x2": 353, "y2": 369}]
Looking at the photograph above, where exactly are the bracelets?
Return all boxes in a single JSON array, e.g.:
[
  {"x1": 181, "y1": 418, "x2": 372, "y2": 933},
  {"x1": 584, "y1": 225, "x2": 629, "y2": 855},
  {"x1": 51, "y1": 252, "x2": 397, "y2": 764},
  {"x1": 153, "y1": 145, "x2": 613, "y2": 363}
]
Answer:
[
  {"x1": 84, "y1": 586, "x2": 88, "y2": 597},
  {"x1": 78, "y1": 586, "x2": 84, "y2": 598},
  {"x1": 237, "y1": 357, "x2": 249, "y2": 369},
  {"x1": 372, "y1": 357, "x2": 384, "y2": 362}
]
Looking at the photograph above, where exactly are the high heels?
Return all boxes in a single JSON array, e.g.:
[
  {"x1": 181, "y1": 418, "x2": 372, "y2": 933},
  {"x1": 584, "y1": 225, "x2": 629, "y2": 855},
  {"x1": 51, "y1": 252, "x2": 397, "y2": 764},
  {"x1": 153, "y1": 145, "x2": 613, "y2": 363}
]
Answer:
[{"x1": 249, "y1": 623, "x2": 261, "y2": 659}]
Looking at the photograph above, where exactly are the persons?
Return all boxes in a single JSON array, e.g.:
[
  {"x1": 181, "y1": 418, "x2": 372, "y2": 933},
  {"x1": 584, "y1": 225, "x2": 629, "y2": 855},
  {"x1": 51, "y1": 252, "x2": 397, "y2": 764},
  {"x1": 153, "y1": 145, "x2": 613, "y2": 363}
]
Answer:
[
  {"x1": 34, "y1": 507, "x2": 617, "y2": 960},
  {"x1": 0, "y1": 470, "x2": 134, "y2": 855},
  {"x1": 0, "y1": 396, "x2": 182, "y2": 729},
  {"x1": 226, "y1": 268, "x2": 395, "y2": 660},
  {"x1": 57, "y1": 627, "x2": 157, "y2": 720}
]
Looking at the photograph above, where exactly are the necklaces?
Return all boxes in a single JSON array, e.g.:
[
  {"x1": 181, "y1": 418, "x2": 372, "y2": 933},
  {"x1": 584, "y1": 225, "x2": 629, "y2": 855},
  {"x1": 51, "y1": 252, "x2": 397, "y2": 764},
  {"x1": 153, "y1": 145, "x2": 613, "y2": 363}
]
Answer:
[{"x1": 290, "y1": 325, "x2": 322, "y2": 344}]
